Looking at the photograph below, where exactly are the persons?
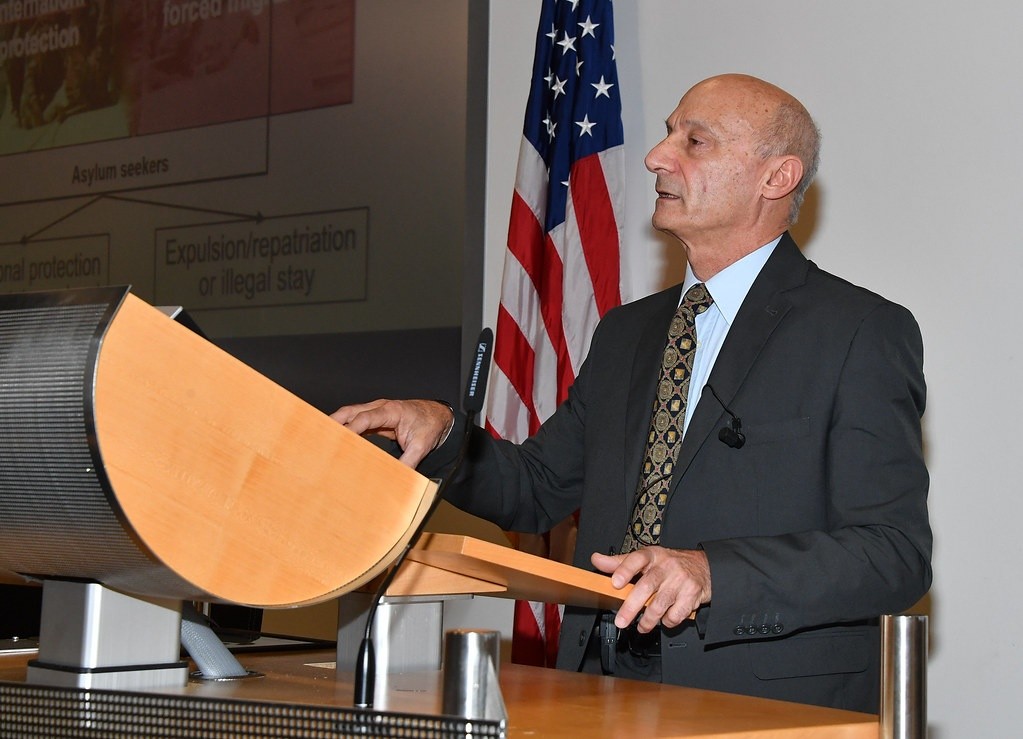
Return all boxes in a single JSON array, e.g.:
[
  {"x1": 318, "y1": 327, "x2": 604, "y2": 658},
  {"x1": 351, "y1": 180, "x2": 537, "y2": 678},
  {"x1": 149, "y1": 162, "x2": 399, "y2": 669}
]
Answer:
[{"x1": 329, "y1": 72, "x2": 933, "y2": 715}]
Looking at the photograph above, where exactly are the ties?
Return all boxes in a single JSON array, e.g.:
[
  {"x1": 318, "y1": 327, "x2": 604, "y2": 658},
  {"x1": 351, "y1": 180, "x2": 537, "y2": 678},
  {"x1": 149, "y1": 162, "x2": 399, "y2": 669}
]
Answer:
[{"x1": 605, "y1": 283, "x2": 714, "y2": 627}]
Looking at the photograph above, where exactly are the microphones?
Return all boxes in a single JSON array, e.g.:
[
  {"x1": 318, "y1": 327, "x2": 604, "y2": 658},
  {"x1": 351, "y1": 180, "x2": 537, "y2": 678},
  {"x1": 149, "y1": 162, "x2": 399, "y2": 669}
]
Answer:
[
  {"x1": 718, "y1": 427, "x2": 740, "y2": 448},
  {"x1": 354, "y1": 327, "x2": 494, "y2": 706}
]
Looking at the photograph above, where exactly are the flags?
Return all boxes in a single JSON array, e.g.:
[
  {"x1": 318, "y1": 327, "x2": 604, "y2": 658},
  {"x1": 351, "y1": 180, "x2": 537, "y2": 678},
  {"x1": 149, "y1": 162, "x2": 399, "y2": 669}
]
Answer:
[{"x1": 484, "y1": 1, "x2": 630, "y2": 667}]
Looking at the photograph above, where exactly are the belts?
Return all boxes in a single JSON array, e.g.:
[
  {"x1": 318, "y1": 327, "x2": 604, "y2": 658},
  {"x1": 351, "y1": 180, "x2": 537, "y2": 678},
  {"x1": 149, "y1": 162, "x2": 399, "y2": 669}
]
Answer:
[{"x1": 592, "y1": 612, "x2": 663, "y2": 658}]
